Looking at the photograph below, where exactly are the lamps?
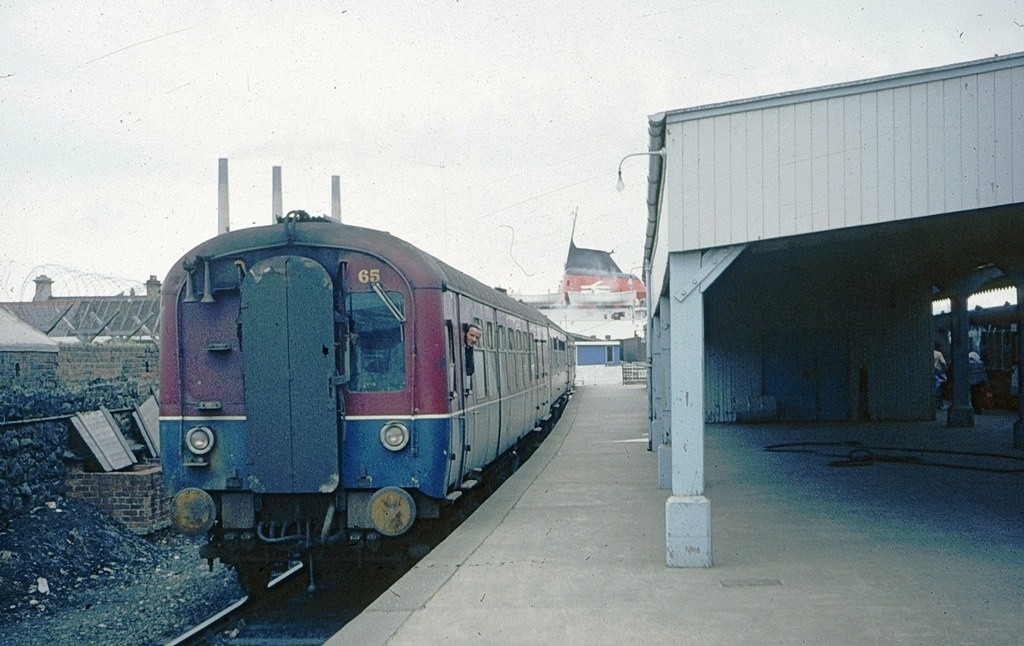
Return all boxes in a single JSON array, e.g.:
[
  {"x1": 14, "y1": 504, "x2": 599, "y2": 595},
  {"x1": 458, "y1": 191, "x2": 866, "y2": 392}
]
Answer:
[
  {"x1": 628, "y1": 266, "x2": 652, "y2": 286},
  {"x1": 616, "y1": 148, "x2": 666, "y2": 192}
]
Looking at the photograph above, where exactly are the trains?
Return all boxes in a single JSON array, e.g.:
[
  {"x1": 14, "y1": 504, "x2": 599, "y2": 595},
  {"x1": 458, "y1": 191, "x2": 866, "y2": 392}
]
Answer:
[{"x1": 156, "y1": 209, "x2": 578, "y2": 613}]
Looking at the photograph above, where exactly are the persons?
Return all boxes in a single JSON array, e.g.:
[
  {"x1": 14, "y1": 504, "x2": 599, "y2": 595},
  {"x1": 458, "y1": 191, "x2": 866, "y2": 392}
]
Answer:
[
  {"x1": 931, "y1": 340, "x2": 949, "y2": 412},
  {"x1": 463, "y1": 324, "x2": 483, "y2": 376},
  {"x1": 968, "y1": 350, "x2": 989, "y2": 415}
]
